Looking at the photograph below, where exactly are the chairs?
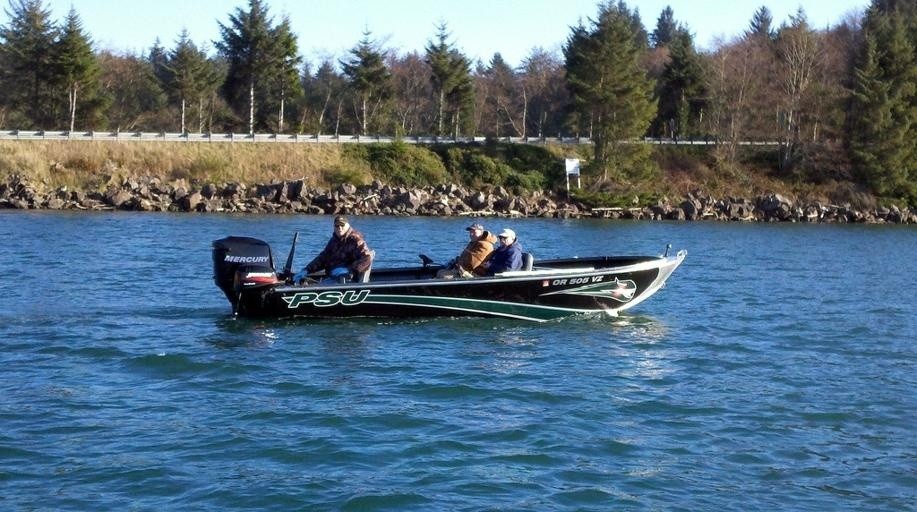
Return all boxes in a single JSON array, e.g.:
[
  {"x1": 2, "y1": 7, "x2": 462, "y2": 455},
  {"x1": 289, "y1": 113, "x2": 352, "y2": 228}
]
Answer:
[{"x1": 522, "y1": 250, "x2": 535, "y2": 272}]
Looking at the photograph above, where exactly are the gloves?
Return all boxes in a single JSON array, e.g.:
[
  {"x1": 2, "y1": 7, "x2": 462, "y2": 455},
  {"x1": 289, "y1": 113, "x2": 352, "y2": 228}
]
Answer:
[
  {"x1": 331, "y1": 268, "x2": 349, "y2": 277},
  {"x1": 292, "y1": 271, "x2": 307, "y2": 282}
]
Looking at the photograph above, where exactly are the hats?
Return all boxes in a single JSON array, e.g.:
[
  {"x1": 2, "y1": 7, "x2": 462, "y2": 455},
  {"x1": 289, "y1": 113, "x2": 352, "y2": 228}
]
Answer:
[
  {"x1": 334, "y1": 215, "x2": 346, "y2": 224},
  {"x1": 498, "y1": 229, "x2": 515, "y2": 240},
  {"x1": 466, "y1": 223, "x2": 483, "y2": 231}
]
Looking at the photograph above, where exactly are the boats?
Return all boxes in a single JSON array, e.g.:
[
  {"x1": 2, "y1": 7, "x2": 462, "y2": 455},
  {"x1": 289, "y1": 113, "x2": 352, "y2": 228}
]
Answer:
[{"x1": 211, "y1": 234, "x2": 687, "y2": 320}]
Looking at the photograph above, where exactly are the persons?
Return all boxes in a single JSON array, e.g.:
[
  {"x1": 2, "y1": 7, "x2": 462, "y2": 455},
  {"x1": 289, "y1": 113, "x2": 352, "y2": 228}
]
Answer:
[
  {"x1": 292, "y1": 214, "x2": 372, "y2": 286},
  {"x1": 473, "y1": 228, "x2": 523, "y2": 277},
  {"x1": 432, "y1": 224, "x2": 497, "y2": 280}
]
